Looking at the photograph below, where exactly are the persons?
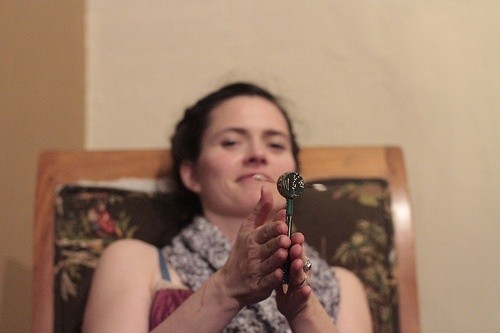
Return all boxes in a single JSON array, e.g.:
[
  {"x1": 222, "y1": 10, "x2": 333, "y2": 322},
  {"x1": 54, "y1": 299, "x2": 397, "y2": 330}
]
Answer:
[{"x1": 80, "y1": 81, "x2": 374, "y2": 333}]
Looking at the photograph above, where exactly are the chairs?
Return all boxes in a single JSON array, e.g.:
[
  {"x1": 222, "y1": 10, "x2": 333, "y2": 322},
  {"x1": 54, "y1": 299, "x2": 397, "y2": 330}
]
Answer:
[{"x1": 32, "y1": 142, "x2": 421, "y2": 333}]
